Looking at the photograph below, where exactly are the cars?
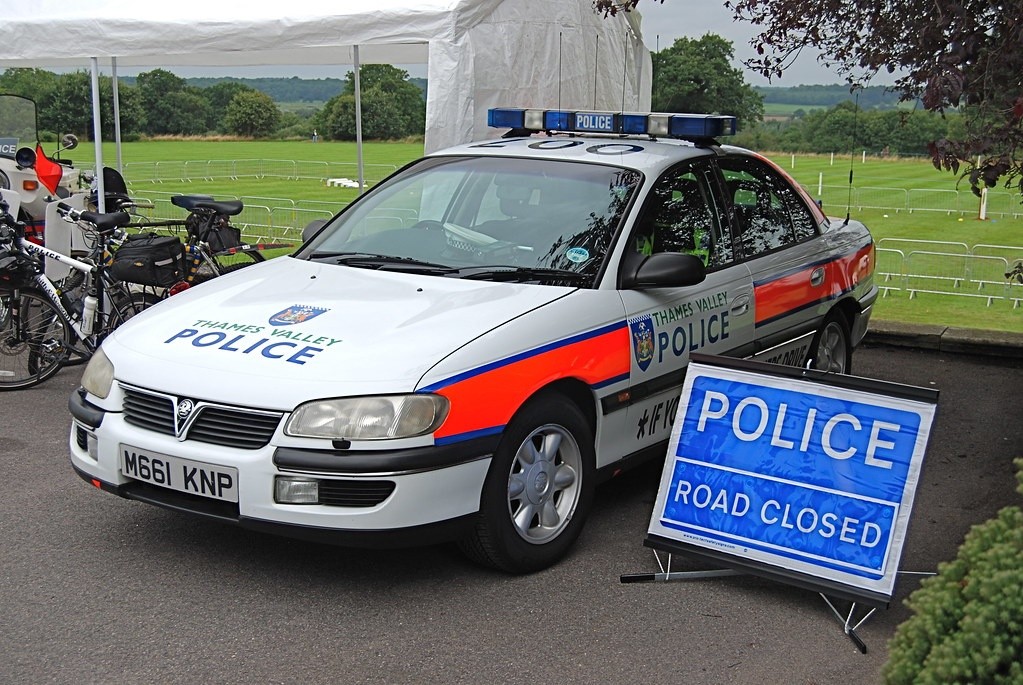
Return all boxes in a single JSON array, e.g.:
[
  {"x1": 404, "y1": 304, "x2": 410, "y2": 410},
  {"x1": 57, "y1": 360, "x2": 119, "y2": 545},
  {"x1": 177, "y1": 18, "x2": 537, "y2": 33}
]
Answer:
[{"x1": 69, "y1": 108, "x2": 879, "y2": 576}]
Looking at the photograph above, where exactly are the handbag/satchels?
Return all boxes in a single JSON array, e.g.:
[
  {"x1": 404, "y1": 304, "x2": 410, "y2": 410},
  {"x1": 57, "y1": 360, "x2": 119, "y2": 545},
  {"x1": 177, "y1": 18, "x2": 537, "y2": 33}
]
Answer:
[
  {"x1": 186, "y1": 212, "x2": 241, "y2": 252},
  {"x1": 111, "y1": 232, "x2": 187, "y2": 287}
]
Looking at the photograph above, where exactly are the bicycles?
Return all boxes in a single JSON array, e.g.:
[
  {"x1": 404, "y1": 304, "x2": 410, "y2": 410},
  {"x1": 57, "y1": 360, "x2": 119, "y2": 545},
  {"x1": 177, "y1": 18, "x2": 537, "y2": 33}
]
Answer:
[{"x1": 0, "y1": 192, "x2": 265, "y2": 390}]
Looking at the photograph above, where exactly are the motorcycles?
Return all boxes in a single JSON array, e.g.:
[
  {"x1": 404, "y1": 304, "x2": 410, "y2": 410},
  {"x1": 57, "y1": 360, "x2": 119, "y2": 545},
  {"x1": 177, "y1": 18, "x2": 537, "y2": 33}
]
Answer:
[{"x1": 0, "y1": 93, "x2": 83, "y2": 331}]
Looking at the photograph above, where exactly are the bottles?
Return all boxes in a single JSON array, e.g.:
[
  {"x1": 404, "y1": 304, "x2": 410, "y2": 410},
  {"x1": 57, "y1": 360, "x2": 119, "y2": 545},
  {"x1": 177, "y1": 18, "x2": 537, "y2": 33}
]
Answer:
[{"x1": 80, "y1": 288, "x2": 98, "y2": 335}]
